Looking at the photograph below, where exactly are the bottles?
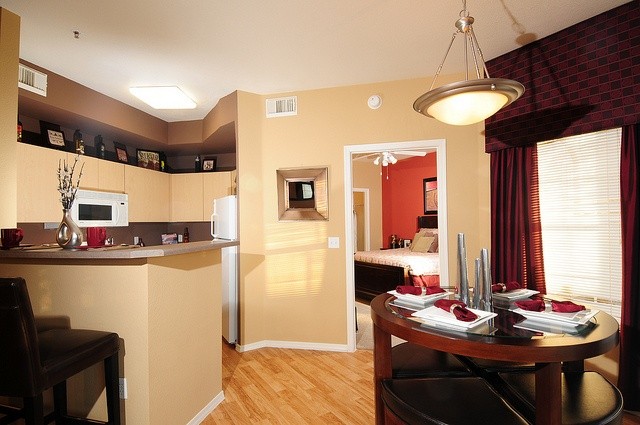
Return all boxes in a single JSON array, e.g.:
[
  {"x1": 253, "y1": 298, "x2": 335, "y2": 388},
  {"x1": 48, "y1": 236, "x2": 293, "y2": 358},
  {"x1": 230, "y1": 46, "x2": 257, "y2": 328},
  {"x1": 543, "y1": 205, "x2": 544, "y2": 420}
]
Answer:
[
  {"x1": 195, "y1": 156, "x2": 199, "y2": 171},
  {"x1": 98, "y1": 138, "x2": 104, "y2": 157},
  {"x1": 184, "y1": 226, "x2": 189, "y2": 242}
]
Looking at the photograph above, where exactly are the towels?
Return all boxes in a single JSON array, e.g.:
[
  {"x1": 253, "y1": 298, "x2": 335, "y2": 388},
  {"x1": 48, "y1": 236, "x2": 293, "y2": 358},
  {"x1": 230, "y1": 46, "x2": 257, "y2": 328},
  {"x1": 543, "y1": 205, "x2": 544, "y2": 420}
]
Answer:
[
  {"x1": 396, "y1": 285, "x2": 446, "y2": 296},
  {"x1": 514, "y1": 298, "x2": 586, "y2": 313},
  {"x1": 493, "y1": 281, "x2": 521, "y2": 293},
  {"x1": 434, "y1": 299, "x2": 478, "y2": 322}
]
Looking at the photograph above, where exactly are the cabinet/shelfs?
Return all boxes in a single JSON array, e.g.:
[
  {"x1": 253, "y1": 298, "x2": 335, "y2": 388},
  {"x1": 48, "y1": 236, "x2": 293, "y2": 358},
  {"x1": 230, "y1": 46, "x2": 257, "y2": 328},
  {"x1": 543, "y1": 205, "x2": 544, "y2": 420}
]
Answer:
[
  {"x1": 67, "y1": 152, "x2": 125, "y2": 194},
  {"x1": 171, "y1": 171, "x2": 236, "y2": 223},
  {"x1": 124, "y1": 163, "x2": 171, "y2": 223},
  {"x1": 17, "y1": 142, "x2": 67, "y2": 223},
  {"x1": 231, "y1": 170, "x2": 238, "y2": 195}
]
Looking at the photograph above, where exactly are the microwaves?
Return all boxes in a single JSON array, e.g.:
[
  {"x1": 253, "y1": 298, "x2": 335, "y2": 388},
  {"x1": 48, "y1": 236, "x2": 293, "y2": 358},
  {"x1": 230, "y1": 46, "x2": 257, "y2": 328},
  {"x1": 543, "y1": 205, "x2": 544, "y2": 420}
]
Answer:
[{"x1": 71, "y1": 189, "x2": 129, "y2": 229}]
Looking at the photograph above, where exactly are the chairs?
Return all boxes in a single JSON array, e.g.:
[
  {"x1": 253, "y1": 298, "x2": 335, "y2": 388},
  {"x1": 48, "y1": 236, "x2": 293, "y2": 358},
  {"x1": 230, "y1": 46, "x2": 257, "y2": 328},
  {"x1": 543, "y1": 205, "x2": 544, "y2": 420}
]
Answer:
[{"x1": 1, "y1": 284, "x2": 121, "y2": 425}]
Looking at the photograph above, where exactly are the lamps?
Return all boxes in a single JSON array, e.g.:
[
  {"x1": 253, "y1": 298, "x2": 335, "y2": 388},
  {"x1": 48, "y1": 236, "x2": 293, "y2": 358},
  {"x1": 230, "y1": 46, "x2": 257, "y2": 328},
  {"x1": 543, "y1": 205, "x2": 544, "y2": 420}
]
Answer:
[
  {"x1": 413, "y1": 0, "x2": 526, "y2": 126},
  {"x1": 352, "y1": 151, "x2": 427, "y2": 161},
  {"x1": 374, "y1": 152, "x2": 397, "y2": 180}
]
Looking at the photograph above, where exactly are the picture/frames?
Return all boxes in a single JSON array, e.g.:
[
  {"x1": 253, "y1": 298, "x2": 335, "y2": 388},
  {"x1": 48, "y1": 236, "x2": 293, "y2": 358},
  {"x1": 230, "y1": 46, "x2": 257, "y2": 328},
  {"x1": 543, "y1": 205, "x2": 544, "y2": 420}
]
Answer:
[
  {"x1": 423, "y1": 177, "x2": 437, "y2": 214},
  {"x1": 202, "y1": 159, "x2": 214, "y2": 172},
  {"x1": 115, "y1": 146, "x2": 128, "y2": 163},
  {"x1": 44, "y1": 128, "x2": 67, "y2": 148}
]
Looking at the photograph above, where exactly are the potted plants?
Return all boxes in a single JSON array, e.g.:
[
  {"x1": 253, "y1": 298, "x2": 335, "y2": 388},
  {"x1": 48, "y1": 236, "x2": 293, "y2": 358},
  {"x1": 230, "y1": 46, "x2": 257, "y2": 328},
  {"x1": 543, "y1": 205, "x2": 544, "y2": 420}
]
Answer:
[{"x1": 54, "y1": 156, "x2": 83, "y2": 249}]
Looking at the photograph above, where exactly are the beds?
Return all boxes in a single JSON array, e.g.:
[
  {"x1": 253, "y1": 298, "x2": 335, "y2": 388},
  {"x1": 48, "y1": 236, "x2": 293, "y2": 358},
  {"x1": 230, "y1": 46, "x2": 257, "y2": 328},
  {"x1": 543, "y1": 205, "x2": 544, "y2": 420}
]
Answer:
[{"x1": 354, "y1": 216, "x2": 438, "y2": 301}]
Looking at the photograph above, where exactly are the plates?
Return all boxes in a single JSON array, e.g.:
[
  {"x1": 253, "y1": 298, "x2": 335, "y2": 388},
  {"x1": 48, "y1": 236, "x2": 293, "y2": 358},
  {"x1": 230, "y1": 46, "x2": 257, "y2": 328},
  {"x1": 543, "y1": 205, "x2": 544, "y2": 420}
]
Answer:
[
  {"x1": 509, "y1": 300, "x2": 600, "y2": 330},
  {"x1": 469, "y1": 286, "x2": 543, "y2": 305},
  {"x1": 388, "y1": 289, "x2": 456, "y2": 308},
  {"x1": 73, "y1": 245, "x2": 112, "y2": 251},
  {"x1": 411, "y1": 303, "x2": 498, "y2": 331}
]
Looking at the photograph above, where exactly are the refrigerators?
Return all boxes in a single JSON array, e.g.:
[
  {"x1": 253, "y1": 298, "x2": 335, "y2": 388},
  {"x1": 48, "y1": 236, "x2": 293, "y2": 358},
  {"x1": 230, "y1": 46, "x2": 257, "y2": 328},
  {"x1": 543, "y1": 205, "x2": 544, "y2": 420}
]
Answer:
[{"x1": 210, "y1": 194, "x2": 238, "y2": 345}]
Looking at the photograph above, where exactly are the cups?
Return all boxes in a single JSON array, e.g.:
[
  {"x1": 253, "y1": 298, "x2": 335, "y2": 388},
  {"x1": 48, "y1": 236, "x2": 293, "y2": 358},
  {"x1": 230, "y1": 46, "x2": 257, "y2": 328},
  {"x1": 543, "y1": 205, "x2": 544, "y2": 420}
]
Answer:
[
  {"x1": 86, "y1": 226, "x2": 106, "y2": 246},
  {"x1": 1, "y1": 228, "x2": 24, "y2": 248}
]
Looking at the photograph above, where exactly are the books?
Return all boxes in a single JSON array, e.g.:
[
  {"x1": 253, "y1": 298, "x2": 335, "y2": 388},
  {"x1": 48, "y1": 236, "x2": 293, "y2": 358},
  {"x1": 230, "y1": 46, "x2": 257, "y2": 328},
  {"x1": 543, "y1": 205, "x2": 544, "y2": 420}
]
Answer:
[
  {"x1": 386, "y1": 284, "x2": 455, "y2": 303},
  {"x1": 508, "y1": 303, "x2": 601, "y2": 326},
  {"x1": 492, "y1": 287, "x2": 541, "y2": 299},
  {"x1": 407, "y1": 303, "x2": 499, "y2": 334}
]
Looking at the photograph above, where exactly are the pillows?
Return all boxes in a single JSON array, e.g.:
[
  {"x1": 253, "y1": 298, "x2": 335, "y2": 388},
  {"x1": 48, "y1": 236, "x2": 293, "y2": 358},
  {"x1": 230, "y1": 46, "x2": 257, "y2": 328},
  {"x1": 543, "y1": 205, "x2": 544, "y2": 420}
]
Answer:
[
  {"x1": 411, "y1": 233, "x2": 433, "y2": 250},
  {"x1": 412, "y1": 237, "x2": 435, "y2": 252},
  {"x1": 419, "y1": 229, "x2": 438, "y2": 234},
  {"x1": 424, "y1": 232, "x2": 438, "y2": 252}
]
